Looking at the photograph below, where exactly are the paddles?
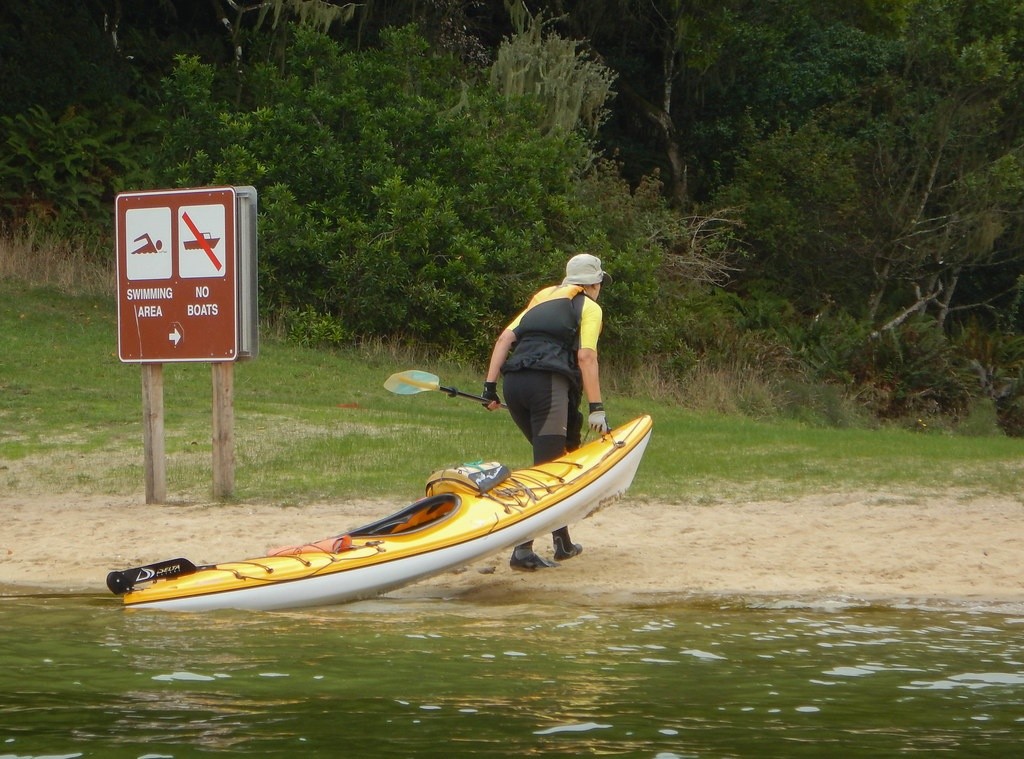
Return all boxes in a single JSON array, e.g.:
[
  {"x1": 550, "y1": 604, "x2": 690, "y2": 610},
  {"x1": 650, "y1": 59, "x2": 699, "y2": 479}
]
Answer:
[{"x1": 381, "y1": 368, "x2": 511, "y2": 410}]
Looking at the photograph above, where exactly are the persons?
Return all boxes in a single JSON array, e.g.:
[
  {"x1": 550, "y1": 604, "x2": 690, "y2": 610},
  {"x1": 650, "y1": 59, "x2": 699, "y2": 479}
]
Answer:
[{"x1": 481, "y1": 253, "x2": 613, "y2": 571}]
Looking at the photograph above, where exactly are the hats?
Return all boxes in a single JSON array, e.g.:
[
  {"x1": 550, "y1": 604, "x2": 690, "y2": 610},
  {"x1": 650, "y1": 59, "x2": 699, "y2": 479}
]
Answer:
[{"x1": 562, "y1": 253, "x2": 612, "y2": 286}]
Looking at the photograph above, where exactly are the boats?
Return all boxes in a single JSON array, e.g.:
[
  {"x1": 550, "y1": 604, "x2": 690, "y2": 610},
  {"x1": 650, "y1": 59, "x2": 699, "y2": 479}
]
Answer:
[{"x1": 105, "y1": 414, "x2": 654, "y2": 608}]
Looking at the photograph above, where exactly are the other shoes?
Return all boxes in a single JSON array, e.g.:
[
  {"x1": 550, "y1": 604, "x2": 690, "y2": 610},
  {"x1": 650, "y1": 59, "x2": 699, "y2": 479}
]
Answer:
[
  {"x1": 509, "y1": 551, "x2": 550, "y2": 572},
  {"x1": 553, "y1": 540, "x2": 582, "y2": 561}
]
